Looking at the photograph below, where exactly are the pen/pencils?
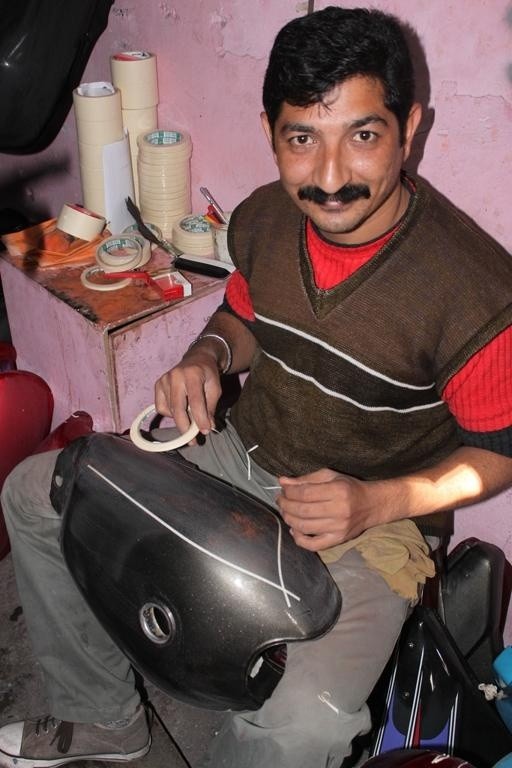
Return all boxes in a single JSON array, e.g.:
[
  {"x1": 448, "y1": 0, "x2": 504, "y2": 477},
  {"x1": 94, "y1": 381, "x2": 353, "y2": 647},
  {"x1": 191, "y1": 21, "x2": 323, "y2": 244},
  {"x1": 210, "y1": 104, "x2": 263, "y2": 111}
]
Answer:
[{"x1": 200, "y1": 186, "x2": 229, "y2": 226}]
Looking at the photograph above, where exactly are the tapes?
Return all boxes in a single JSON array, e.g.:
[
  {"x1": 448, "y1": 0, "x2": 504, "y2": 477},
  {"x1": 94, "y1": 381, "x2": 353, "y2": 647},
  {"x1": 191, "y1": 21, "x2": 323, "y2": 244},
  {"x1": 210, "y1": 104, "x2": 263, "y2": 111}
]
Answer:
[
  {"x1": 130, "y1": 401, "x2": 200, "y2": 453},
  {"x1": 56, "y1": 51, "x2": 214, "y2": 291}
]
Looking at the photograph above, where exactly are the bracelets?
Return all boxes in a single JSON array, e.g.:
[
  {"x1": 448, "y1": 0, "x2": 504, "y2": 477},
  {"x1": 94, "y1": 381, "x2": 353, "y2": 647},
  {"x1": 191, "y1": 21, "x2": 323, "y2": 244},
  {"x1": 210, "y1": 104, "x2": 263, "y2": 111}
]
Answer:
[{"x1": 189, "y1": 334, "x2": 237, "y2": 381}]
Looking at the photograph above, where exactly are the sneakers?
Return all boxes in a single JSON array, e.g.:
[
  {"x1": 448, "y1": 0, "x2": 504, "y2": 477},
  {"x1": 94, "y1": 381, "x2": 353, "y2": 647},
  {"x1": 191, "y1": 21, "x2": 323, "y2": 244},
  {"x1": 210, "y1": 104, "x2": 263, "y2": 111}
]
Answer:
[{"x1": 0, "y1": 706, "x2": 151, "y2": 768}]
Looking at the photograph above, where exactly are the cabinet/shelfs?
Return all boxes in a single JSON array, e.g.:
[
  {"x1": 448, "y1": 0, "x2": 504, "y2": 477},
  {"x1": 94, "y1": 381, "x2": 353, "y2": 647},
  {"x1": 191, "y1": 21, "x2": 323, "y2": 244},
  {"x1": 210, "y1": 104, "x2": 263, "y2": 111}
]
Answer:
[{"x1": 1, "y1": 218, "x2": 233, "y2": 434}]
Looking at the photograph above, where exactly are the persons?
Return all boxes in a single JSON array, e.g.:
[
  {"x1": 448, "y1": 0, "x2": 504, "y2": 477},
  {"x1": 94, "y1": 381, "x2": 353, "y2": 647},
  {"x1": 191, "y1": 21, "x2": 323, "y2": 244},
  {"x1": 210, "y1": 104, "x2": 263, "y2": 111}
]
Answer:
[{"x1": 2, "y1": 6, "x2": 511, "y2": 768}]
